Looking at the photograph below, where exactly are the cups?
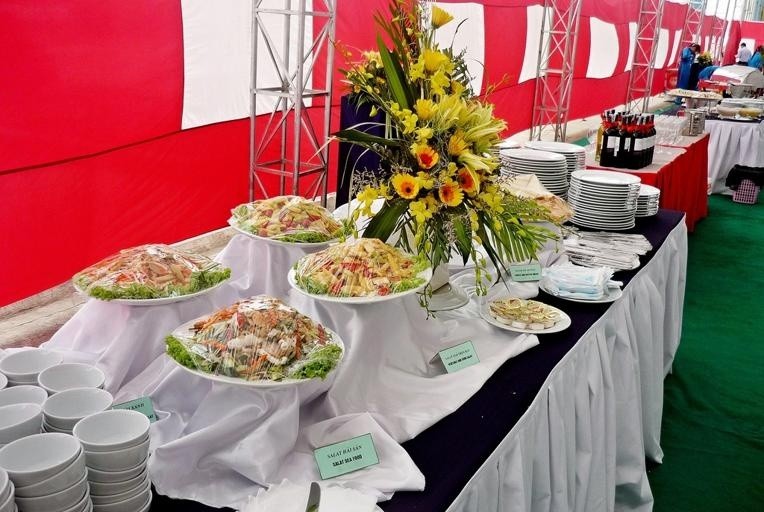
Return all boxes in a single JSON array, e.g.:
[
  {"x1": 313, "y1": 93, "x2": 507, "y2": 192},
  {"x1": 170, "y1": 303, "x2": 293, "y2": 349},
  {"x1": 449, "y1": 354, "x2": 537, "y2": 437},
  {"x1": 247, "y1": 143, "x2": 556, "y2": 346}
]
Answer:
[{"x1": 643, "y1": 112, "x2": 689, "y2": 148}]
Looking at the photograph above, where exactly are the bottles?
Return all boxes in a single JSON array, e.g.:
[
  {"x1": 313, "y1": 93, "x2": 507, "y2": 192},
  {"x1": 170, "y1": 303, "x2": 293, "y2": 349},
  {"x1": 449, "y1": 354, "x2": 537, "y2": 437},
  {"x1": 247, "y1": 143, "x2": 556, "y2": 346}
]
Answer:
[{"x1": 595, "y1": 108, "x2": 657, "y2": 170}]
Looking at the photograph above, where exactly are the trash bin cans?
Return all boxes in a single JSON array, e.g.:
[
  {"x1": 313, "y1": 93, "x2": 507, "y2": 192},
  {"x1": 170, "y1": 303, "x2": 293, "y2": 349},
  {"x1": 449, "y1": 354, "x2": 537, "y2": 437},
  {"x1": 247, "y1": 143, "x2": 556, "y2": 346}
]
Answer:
[{"x1": 725, "y1": 164, "x2": 760, "y2": 204}]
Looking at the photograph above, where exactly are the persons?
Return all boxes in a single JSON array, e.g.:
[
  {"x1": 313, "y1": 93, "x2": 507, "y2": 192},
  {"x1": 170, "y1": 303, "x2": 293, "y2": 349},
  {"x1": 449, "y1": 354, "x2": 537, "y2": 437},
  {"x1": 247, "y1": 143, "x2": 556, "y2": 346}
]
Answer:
[
  {"x1": 674, "y1": 43, "x2": 701, "y2": 104},
  {"x1": 734, "y1": 42, "x2": 751, "y2": 67},
  {"x1": 748, "y1": 45, "x2": 764, "y2": 69}
]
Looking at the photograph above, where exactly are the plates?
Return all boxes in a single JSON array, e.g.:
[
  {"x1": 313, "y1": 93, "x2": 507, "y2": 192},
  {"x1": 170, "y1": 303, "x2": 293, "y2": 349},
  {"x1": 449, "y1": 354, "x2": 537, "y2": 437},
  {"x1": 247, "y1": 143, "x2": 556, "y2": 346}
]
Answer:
[
  {"x1": 288, "y1": 264, "x2": 433, "y2": 304},
  {"x1": 226, "y1": 217, "x2": 346, "y2": 249},
  {"x1": 417, "y1": 283, "x2": 470, "y2": 311},
  {"x1": 490, "y1": 146, "x2": 522, "y2": 164},
  {"x1": 165, "y1": 314, "x2": 344, "y2": 387},
  {"x1": 538, "y1": 276, "x2": 622, "y2": 303},
  {"x1": 566, "y1": 170, "x2": 641, "y2": 231},
  {"x1": 524, "y1": 141, "x2": 586, "y2": 182},
  {"x1": 74, "y1": 278, "x2": 231, "y2": 307},
  {"x1": 498, "y1": 149, "x2": 570, "y2": 224},
  {"x1": 479, "y1": 297, "x2": 571, "y2": 335},
  {"x1": 635, "y1": 184, "x2": 661, "y2": 218}
]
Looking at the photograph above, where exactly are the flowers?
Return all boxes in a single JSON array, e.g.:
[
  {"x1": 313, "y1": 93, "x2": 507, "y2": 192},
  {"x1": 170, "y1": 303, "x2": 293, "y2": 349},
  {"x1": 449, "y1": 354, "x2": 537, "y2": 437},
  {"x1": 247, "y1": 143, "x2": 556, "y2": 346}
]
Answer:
[{"x1": 324, "y1": 0, "x2": 580, "y2": 320}]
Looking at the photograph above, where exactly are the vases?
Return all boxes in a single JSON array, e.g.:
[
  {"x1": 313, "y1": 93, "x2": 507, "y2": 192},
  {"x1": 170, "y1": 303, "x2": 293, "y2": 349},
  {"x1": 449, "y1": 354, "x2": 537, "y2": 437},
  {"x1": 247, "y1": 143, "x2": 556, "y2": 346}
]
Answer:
[{"x1": 402, "y1": 220, "x2": 449, "y2": 295}]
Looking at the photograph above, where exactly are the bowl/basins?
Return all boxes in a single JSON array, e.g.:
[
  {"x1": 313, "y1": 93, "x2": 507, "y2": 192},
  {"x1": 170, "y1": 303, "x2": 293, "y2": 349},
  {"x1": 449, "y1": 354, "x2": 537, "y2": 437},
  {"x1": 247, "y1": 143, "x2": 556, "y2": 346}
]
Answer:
[
  {"x1": 37, "y1": 362, "x2": 106, "y2": 394},
  {"x1": 0, "y1": 432, "x2": 93, "y2": 512},
  {"x1": 0, "y1": 467, "x2": 18, "y2": 512},
  {"x1": 0, "y1": 372, "x2": 8, "y2": 390},
  {"x1": 39, "y1": 386, "x2": 114, "y2": 434},
  {"x1": 0, "y1": 350, "x2": 63, "y2": 385},
  {"x1": 0, "y1": 401, "x2": 43, "y2": 441},
  {"x1": 71, "y1": 409, "x2": 154, "y2": 512},
  {"x1": 0, "y1": 384, "x2": 47, "y2": 407}
]
